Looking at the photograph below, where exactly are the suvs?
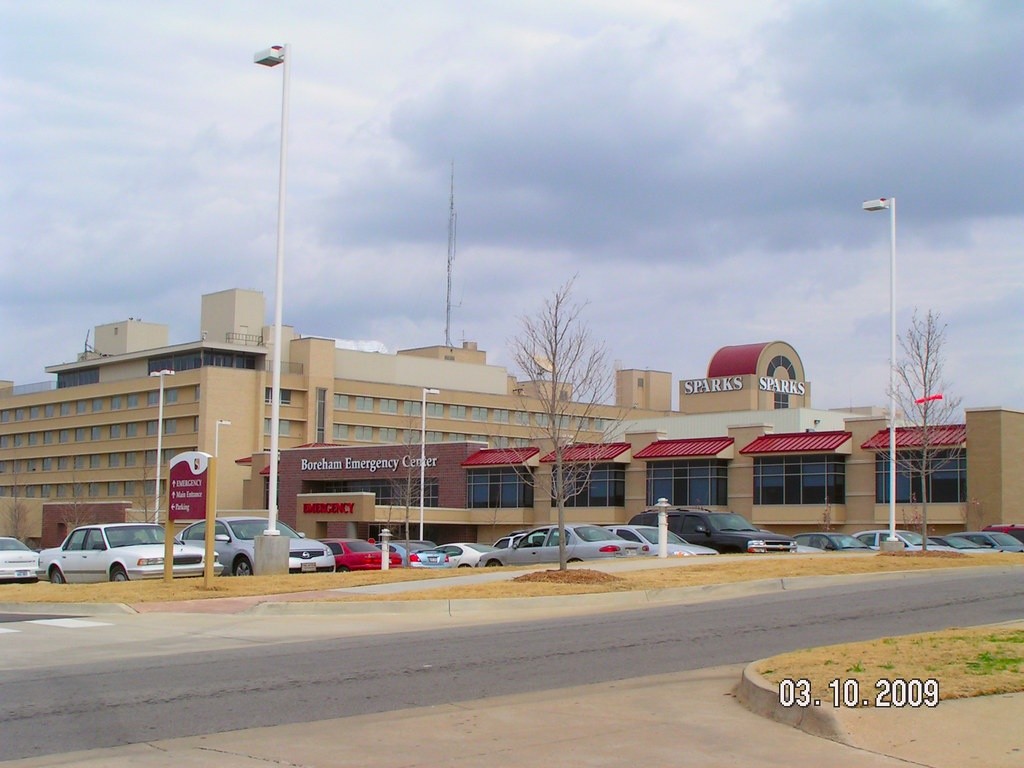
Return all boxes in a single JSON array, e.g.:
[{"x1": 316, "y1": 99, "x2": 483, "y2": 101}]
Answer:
[{"x1": 628, "y1": 506, "x2": 799, "y2": 553}]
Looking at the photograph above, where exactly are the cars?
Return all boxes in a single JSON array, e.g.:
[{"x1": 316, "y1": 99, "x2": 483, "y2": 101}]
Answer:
[
  {"x1": 39, "y1": 522, "x2": 225, "y2": 585},
  {"x1": 315, "y1": 538, "x2": 403, "y2": 574},
  {"x1": 914, "y1": 535, "x2": 1000, "y2": 553},
  {"x1": 602, "y1": 525, "x2": 719, "y2": 557},
  {"x1": 374, "y1": 530, "x2": 548, "y2": 570},
  {"x1": 163, "y1": 516, "x2": 336, "y2": 577},
  {"x1": 0, "y1": 537, "x2": 43, "y2": 582},
  {"x1": 981, "y1": 524, "x2": 1024, "y2": 543},
  {"x1": 841, "y1": 530, "x2": 959, "y2": 555},
  {"x1": 948, "y1": 532, "x2": 1024, "y2": 554},
  {"x1": 478, "y1": 524, "x2": 650, "y2": 569},
  {"x1": 791, "y1": 532, "x2": 873, "y2": 552}
]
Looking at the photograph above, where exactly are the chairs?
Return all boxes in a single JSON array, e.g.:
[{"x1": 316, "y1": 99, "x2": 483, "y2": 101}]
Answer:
[
  {"x1": 827, "y1": 539, "x2": 843, "y2": 549},
  {"x1": 812, "y1": 539, "x2": 821, "y2": 548},
  {"x1": 215, "y1": 526, "x2": 225, "y2": 535},
  {"x1": 866, "y1": 537, "x2": 875, "y2": 546},
  {"x1": 194, "y1": 532, "x2": 204, "y2": 539}
]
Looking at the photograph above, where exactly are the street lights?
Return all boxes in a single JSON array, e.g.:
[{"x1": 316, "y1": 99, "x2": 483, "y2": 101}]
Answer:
[
  {"x1": 862, "y1": 195, "x2": 906, "y2": 551},
  {"x1": 149, "y1": 369, "x2": 176, "y2": 523},
  {"x1": 215, "y1": 420, "x2": 232, "y2": 457},
  {"x1": 418, "y1": 387, "x2": 440, "y2": 540},
  {"x1": 252, "y1": 42, "x2": 293, "y2": 577}
]
